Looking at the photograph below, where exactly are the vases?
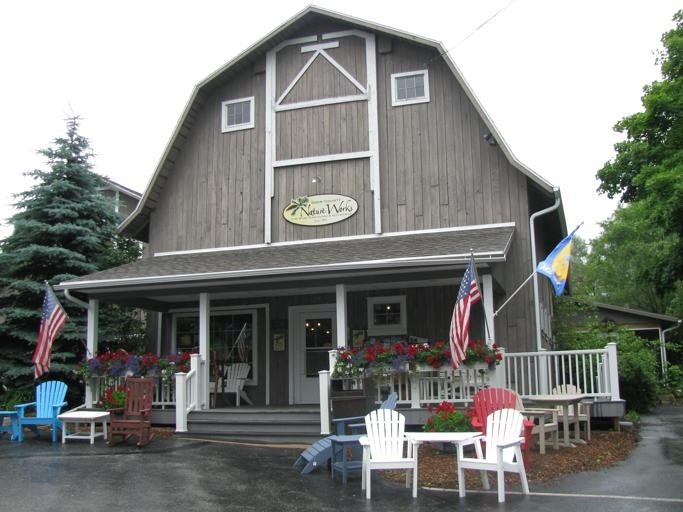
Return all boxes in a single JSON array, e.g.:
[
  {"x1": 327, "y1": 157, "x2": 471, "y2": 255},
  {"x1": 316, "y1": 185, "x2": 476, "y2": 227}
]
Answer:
[{"x1": 429, "y1": 440, "x2": 475, "y2": 454}]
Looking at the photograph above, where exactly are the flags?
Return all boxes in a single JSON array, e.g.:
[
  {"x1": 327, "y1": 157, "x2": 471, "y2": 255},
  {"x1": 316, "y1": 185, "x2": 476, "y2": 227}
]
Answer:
[
  {"x1": 535, "y1": 225, "x2": 578, "y2": 297},
  {"x1": 236, "y1": 325, "x2": 248, "y2": 364},
  {"x1": 31, "y1": 283, "x2": 68, "y2": 379},
  {"x1": 449, "y1": 257, "x2": 481, "y2": 370}
]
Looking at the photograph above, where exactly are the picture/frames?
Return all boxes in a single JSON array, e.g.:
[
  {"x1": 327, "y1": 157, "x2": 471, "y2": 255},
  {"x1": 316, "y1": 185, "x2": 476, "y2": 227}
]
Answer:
[
  {"x1": 272, "y1": 331, "x2": 288, "y2": 354},
  {"x1": 349, "y1": 327, "x2": 367, "y2": 347}
]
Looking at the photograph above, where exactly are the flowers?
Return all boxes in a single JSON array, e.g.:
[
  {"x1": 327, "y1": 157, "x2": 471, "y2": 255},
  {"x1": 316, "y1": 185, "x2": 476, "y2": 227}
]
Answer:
[{"x1": 421, "y1": 399, "x2": 477, "y2": 432}]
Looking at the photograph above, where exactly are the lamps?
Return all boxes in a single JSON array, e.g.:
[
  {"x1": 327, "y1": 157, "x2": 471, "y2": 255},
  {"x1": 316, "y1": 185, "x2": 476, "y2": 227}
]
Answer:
[{"x1": 310, "y1": 175, "x2": 322, "y2": 183}]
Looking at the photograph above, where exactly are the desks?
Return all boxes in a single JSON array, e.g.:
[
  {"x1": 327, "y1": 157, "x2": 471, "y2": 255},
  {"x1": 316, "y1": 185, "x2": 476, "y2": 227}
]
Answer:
[
  {"x1": 516, "y1": 392, "x2": 587, "y2": 450},
  {"x1": 398, "y1": 430, "x2": 491, "y2": 499},
  {"x1": 56, "y1": 410, "x2": 111, "y2": 444},
  {"x1": 329, "y1": 435, "x2": 366, "y2": 484},
  {"x1": 0, "y1": 409, "x2": 16, "y2": 442}
]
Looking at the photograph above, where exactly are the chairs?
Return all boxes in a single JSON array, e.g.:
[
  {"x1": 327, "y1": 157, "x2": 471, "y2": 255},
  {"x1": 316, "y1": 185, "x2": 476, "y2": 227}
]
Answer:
[
  {"x1": 356, "y1": 407, "x2": 420, "y2": 501},
  {"x1": 326, "y1": 390, "x2": 399, "y2": 488},
  {"x1": 208, "y1": 363, "x2": 219, "y2": 409},
  {"x1": 211, "y1": 361, "x2": 255, "y2": 408},
  {"x1": 455, "y1": 407, "x2": 530, "y2": 504},
  {"x1": 16, "y1": 379, "x2": 68, "y2": 444},
  {"x1": 511, "y1": 388, "x2": 560, "y2": 455},
  {"x1": 103, "y1": 375, "x2": 155, "y2": 447},
  {"x1": 548, "y1": 383, "x2": 588, "y2": 443},
  {"x1": 468, "y1": 386, "x2": 535, "y2": 473}
]
